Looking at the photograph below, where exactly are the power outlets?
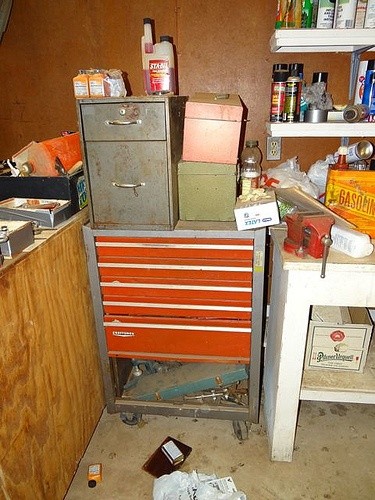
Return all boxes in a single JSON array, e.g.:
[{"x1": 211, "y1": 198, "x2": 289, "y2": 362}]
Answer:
[{"x1": 266, "y1": 136, "x2": 281, "y2": 160}]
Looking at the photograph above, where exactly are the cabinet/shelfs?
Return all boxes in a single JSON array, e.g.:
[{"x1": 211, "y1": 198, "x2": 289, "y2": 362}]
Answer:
[
  {"x1": 73, "y1": 93, "x2": 176, "y2": 233},
  {"x1": 269, "y1": 29, "x2": 375, "y2": 140},
  {"x1": 86, "y1": 223, "x2": 266, "y2": 444}
]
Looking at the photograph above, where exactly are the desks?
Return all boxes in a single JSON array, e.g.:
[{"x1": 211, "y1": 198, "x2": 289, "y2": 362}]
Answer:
[
  {"x1": 0, "y1": 210, "x2": 132, "y2": 500},
  {"x1": 267, "y1": 224, "x2": 375, "y2": 463}
]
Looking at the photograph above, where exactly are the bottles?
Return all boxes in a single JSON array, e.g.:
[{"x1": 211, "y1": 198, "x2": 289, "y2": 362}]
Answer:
[
  {"x1": 333, "y1": 145, "x2": 349, "y2": 170},
  {"x1": 330, "y1": 225, "x2": 374, "y2": 259},
  {"x1": 332, "y1": 140, "x2": 373, "y2": 164},
  {"x1": 275, "y1": 0, "x2": 375, "y2": 29},
  {"x1": 73, "y1": 69, "x2": 105, "y2": 99},
  {"x1": 239, "y1": 139, "x2": 262, "y2": 196},
  {"x1": 270, "y1": 63, "x2": 328, "y2": 123},
  {"x1": 343, "y1": 59, "x2": 375, "y2": 123}
]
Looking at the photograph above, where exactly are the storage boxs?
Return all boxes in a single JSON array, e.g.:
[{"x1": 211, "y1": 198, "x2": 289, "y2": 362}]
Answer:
[
  {"x1": 304, "y1": 305, "x2": 373, "y2": 374},
  {"x1": 233, "y1": 191, "x2": 280, "y2": 230},
  {"x1": 0, "y1": 164, "x2": 88, "y2": 213},
  {"x1": 0, "y1": 220, "x2": 34, "y2": 258},
  {"x1": 177, "y1": 160, "x2": 236, "y2": 222},
  {"x1": 0, "y1": 197, "x2": 72, "y2": 228},
  {"x1": 181, "y1": 93, "x2": 240, "y2": 164},
  {"x1": 324, "y1": 169, "x2": 375, "y2": 238}
]
